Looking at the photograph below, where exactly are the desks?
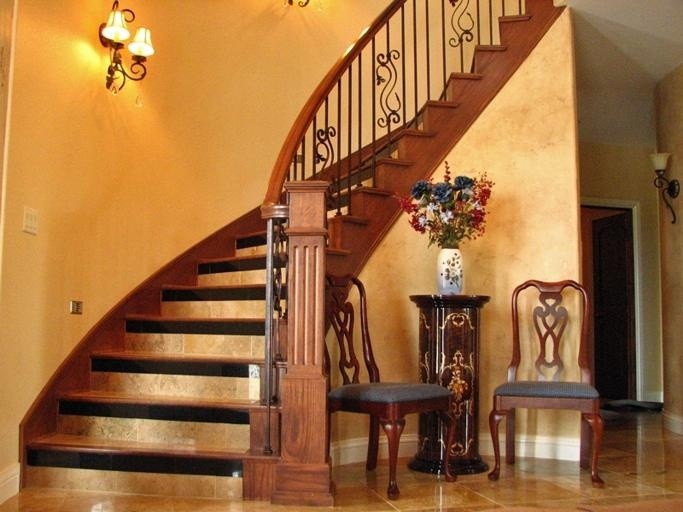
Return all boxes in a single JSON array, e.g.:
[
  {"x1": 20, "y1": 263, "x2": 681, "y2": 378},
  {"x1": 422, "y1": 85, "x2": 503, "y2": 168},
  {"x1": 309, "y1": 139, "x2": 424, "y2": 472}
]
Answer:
[{"x1": 408, "y1": 294, "x2": 492, "y2": 476}]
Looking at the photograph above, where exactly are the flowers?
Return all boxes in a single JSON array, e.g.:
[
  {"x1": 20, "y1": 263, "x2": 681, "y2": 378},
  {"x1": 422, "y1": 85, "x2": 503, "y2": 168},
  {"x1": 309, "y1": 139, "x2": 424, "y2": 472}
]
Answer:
[{"x1": 391, "y1": 160, "x2": 497, "y2": 250}]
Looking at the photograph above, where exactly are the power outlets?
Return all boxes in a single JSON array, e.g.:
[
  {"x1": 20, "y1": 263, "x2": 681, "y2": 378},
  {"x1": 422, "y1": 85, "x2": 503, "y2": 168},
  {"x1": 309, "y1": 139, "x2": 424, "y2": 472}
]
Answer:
[{"x1": 69, "y1": 299, "x2": 84, "y2": 316}]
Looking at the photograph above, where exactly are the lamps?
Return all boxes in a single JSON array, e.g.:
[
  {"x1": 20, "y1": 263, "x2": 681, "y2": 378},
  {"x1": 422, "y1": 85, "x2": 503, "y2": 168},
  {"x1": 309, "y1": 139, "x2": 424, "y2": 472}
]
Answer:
[
  {"x1": 99, "y1": 1, "x2": 155, "y2": 95},
  {"x1": 648, "y1": 152, "x2": 679, "y2": 223}
]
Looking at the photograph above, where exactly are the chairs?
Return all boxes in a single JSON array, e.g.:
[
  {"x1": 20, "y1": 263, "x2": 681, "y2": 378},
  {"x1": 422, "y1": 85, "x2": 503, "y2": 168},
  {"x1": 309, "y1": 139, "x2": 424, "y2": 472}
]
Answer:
[
  {"x1": 322, "y1": 271, "x2": 459, "y2": 502},
  {"x1": 487, "y1": 279, "x2": 606, "y2": 490}
]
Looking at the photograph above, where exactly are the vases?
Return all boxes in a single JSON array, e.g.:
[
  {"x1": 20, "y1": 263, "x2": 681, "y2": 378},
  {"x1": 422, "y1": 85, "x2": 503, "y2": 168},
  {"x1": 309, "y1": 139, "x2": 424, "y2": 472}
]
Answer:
[{"x1": 435, "y1": 247, "x2": 464, "y2": 296}]
